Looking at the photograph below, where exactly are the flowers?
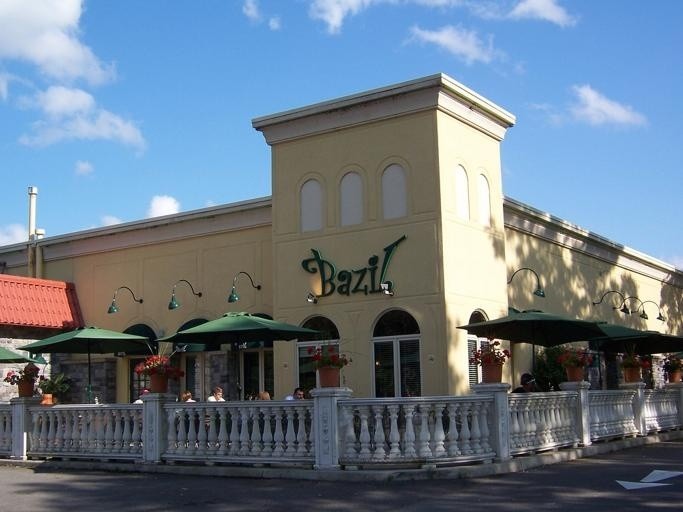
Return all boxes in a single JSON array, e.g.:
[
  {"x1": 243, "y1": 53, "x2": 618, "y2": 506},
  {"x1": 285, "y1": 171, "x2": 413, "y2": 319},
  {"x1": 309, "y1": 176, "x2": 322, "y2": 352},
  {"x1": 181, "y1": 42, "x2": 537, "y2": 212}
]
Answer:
[
  {"x1": 307, "y1": 338, "x2": 353, "y2": 370},
  {"x1": 468, "y1": 331, "x2": 511, "y2": 367},
  {"x1": 132, "y1": 355, "x2": 185, "y2": 378},
  {"x1": 4, "y1": 362, "x2": 39, "y2": 385},
  {"x1": 549, "y1": 344, "x2": 682, "y2": 371}
]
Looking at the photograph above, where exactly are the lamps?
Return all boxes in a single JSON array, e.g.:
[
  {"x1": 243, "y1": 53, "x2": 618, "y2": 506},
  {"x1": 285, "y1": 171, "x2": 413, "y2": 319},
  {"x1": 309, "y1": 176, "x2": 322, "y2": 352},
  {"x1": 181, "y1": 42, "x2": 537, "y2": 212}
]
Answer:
[
  {"x1": 507, "y1": 266, "x2": 545, "y2": 299},
  {"x1": 592, "y1": 290, "x2": 666, "y2": 323},
  {"x1": 106, "y1": 270, "x2": 261, "y2": 315}
]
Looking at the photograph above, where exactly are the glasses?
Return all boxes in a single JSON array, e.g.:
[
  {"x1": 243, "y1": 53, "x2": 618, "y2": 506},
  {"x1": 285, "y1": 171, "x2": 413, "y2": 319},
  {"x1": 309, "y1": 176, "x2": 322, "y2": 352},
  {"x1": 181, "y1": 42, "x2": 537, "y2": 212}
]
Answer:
[
  {"x1": 296, "y1": 394, "x2": 304, "y2": 395},
  {"x1": 527, "y1": 379, "x2": 535, "y2": 384}
]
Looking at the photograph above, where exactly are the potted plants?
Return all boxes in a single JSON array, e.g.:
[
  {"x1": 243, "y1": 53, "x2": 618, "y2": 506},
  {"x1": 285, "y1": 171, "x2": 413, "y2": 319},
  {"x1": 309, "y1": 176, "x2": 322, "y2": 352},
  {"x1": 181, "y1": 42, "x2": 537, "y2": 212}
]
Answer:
[{"x1": 37, "y1": 372, "x2": 72, "y2": 405}]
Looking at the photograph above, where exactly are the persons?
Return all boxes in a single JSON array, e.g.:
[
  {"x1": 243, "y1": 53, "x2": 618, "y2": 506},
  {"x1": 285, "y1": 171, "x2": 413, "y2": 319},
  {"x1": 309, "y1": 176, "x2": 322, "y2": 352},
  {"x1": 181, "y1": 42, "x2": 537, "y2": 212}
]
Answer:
[{"x1": 510, "y1": 373, "x2": 538, "y2": 393}]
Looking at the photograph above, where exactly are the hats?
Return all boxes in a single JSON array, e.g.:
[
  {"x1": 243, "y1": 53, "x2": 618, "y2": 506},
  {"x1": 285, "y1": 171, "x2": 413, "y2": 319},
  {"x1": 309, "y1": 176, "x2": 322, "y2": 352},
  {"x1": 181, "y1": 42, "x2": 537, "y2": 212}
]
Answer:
[{"x1": 521, "y1": 374, "x2": 533, "y2": 385}]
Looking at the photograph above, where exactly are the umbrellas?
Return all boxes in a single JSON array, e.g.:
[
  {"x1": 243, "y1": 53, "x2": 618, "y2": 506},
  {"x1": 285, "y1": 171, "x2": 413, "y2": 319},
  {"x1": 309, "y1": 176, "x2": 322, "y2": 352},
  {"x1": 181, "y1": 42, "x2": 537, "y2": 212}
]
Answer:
[{"x1": 456, "y1": 308, "x2": 682, "y2": 388}]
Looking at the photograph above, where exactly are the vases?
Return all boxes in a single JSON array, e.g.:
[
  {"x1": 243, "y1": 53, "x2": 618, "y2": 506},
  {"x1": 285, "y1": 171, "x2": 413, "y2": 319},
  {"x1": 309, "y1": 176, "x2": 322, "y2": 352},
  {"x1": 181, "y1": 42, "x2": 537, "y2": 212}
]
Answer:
[
  {"x1": 148, "y1": 372, "x2": 168, "y2": 393},
  {"x1": 16, "y1": 379, "x2": 32, "y2": 397},
  {"x1": 564, "y1": 365, "x2": 682, "y2": 384},
  {"x1": 316, "y1": 365, "x2": 342, "y2": 386},
  {"x1": 480, "y1": 362, "x2": 503, "y2": 384}
]
